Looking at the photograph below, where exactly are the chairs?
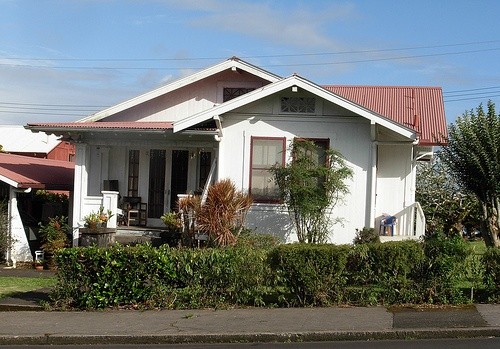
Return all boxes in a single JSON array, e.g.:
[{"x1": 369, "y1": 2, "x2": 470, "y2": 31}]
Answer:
[{"x1": 117, "y1": 193, "x2": 149, "y2": 228}]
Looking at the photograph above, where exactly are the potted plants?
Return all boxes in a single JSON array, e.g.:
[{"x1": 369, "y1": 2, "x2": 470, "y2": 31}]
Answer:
[
  {"x1": 83, "y1": 206, "x2": 114, "y2": 228},
  {"x1": 34, "y1": 259, "x2": 45, "y2": 270}
]
